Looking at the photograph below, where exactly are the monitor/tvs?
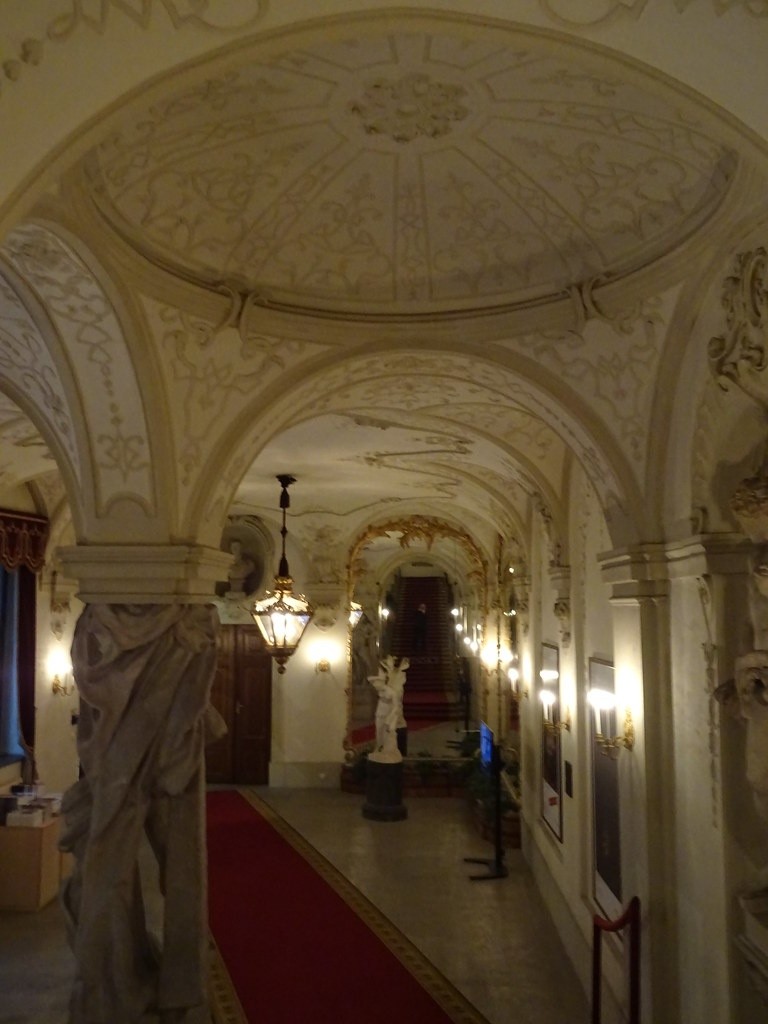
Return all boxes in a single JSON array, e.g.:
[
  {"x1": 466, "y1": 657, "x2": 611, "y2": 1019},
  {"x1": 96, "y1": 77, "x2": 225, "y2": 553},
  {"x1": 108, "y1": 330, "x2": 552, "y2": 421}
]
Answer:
[{"x1": 479, "y1": 720, "x2": 494, "y2": 769}]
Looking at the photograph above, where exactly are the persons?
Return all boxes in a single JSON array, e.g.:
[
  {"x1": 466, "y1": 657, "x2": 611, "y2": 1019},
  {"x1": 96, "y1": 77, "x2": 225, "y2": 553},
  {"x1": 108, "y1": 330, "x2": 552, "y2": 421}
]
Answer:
[{"x1": 367, "y1": 676, "x2": 398, "y2": 751}]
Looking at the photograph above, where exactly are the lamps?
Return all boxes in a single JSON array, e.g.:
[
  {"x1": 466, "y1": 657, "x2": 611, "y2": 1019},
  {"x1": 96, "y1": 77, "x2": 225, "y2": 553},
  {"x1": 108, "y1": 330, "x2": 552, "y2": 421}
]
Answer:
[
  {"x1": 53, "y1": 665, "x2": 75, "y2": 696},
  {"x1": 349, "y1": 601, "x2": 364, "y2": 628},
  {"x1": 481, "y1": 646, "x2": 635, "y2": 760},
  {"x1": 248, "y1": 475, "x2": 315, "y2": 675}
]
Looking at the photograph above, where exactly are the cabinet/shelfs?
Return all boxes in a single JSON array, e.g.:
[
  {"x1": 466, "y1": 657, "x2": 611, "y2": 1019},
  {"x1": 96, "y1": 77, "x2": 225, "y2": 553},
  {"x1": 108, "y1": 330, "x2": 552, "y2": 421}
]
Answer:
[{"x1": 0, "y1": 814, "x2": 76, "y2": 912}]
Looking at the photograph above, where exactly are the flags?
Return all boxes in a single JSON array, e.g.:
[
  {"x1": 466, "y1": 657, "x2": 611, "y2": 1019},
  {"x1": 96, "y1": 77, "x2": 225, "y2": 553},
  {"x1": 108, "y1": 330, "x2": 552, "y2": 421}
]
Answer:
[{"x1": 480, "y1": 723, "x2": 492, "y2": 767}]
